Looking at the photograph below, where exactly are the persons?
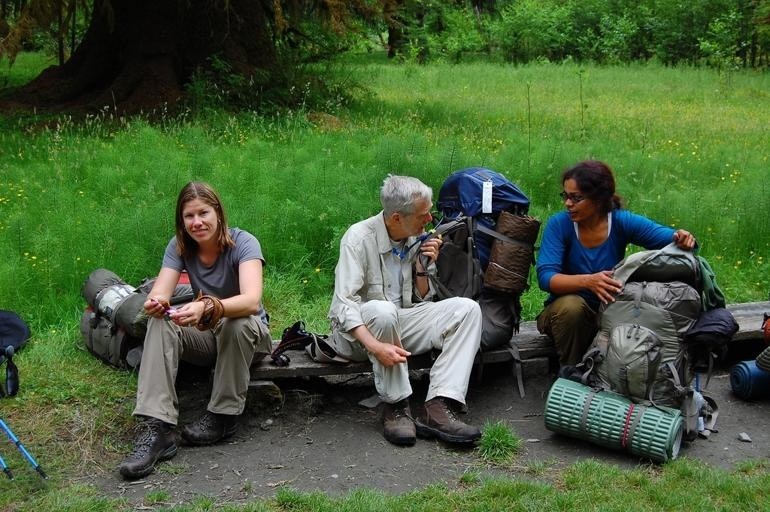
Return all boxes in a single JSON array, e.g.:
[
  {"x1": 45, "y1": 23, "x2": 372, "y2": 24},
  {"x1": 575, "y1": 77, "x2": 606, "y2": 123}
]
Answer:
[
  {"x1": 116, "y1": 180, "x2": 274, "y2": 482},
  {"x1": 533, "y1": 158, "x2": 698, "y2": 380},
  {"x1": 327, "y1": 174, "x2": 484, "y2": 447}
]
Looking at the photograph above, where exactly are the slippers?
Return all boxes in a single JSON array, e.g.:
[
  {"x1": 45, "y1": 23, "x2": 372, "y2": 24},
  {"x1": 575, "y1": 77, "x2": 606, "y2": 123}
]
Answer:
[
  {"x1": 421, "y1": 167, "x2": 529, "y2": 352},
  {"x1": 579, "y1": 241, "x2": 739, "y2": 441},
  {"x1": 81, "y1": 270, "x2": 194, "y2": 369}
]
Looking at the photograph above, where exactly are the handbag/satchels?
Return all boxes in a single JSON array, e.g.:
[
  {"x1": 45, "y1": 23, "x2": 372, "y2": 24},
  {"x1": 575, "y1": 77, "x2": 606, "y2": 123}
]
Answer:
[{"x1": 414, "y1": 269, "x2": 431, "y2": 278}]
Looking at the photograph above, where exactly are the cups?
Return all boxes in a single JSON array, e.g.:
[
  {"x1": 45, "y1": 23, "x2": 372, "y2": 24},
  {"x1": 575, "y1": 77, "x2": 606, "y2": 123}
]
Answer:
[{"x1": 560, "y1": 190, "x2": 584, "y2": 202}]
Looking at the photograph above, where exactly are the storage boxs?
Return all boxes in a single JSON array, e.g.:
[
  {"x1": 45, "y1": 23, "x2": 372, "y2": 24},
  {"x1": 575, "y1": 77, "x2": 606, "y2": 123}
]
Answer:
[{"x1": 251, "y1": 301, "x2": 770, "y2": 395}]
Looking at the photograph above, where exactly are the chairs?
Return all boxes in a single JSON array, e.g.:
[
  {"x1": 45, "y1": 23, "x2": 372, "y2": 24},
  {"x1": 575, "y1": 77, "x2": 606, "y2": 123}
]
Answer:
[
  {"x1": 414, "y1": 399, "x2": 481, "y2": 443},
  {"x1": 182, "y1": 411, "x2": 239, "y2": 445},
  {"x1": 383, "y1": 399, "x2": 417, "y2": 444},
  {"x1": 120, "y1": 417, "x2": 177, "y2": 477}
]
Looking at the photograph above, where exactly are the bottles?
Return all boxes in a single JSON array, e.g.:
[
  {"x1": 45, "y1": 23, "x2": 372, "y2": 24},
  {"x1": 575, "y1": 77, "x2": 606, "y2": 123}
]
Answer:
[{"x1": 305, "y1": 332, "x2": 350, "y2": 363}]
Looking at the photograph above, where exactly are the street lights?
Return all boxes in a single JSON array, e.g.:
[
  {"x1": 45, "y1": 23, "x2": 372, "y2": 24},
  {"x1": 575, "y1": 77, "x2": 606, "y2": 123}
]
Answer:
[{"x1": 0, "y1": 310, "x2": 29, "y2": 359}]
